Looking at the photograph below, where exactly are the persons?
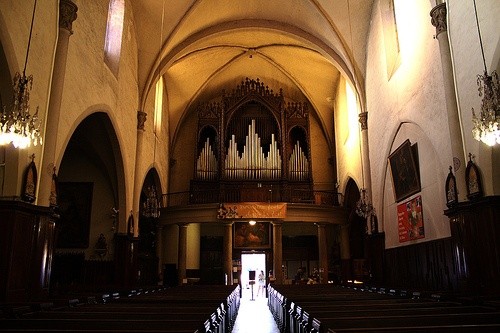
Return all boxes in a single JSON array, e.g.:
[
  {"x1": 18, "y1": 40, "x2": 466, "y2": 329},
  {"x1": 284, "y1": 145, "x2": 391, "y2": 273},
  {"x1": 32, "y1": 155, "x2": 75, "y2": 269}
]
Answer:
[
  {"x1": 296, "y1": 268, "x2": 321, "y2": 285},
  {"x1": 257, "y1": 270, "x2": 274, "y2": 298}
]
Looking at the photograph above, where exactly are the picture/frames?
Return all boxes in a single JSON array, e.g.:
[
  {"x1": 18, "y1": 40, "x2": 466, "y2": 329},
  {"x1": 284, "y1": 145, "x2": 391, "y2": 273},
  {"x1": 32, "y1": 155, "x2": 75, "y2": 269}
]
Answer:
[
  {"x1": 387, "y1": 139, "x2": 420, "y2": 202},
  {"x1": 234, "y1": 221, "x2": 272, "y2": 249}
]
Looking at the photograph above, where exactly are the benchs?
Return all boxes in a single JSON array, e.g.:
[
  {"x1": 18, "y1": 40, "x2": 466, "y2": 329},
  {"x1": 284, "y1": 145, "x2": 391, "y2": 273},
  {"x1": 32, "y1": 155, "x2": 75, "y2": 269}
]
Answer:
[
  {"x1": 267, "y1": 284, "x2": 500, "y2": 333},
  {"x1": 0, "y1": 283, "x2": 241, "y2": 333}
]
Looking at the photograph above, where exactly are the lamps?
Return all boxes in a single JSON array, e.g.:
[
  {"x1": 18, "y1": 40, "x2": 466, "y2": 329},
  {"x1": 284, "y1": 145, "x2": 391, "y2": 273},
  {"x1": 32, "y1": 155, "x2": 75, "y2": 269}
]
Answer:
[
  {"x1": 348, "y1": 0, "x2": 378, "y2": 219},
  {"x1": 0, "y1": 0, "x2": 44, "y2": 150},
  {"x1": 471, "y1": 0, "x2": 500, "y2": 147},
  {"x1": 139, "y1": 0, "x2": 166, "y2": 218}
]
